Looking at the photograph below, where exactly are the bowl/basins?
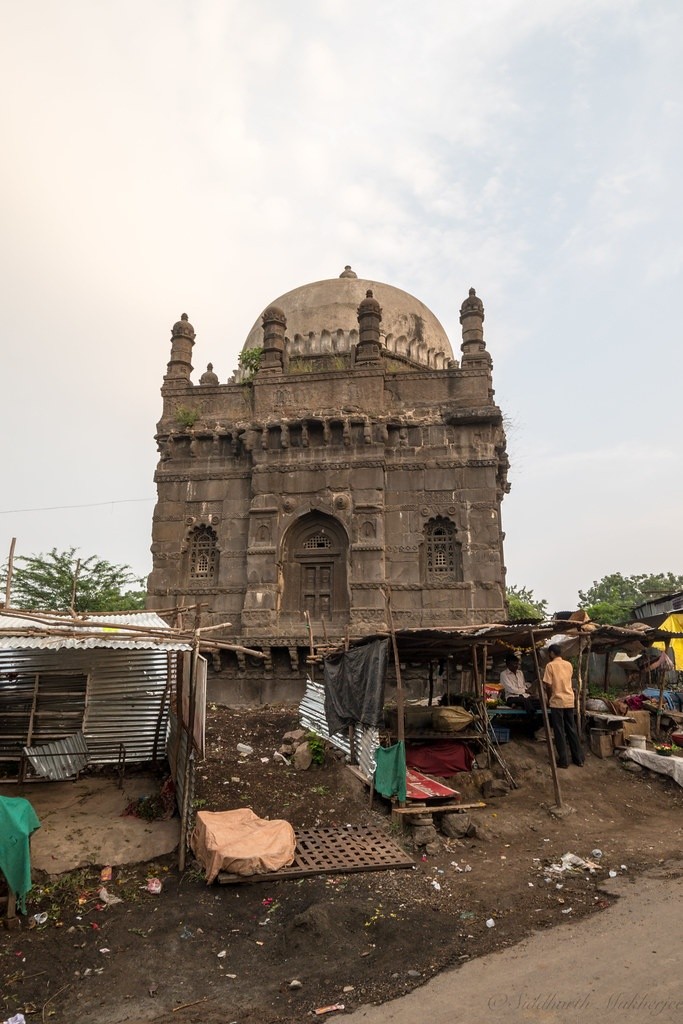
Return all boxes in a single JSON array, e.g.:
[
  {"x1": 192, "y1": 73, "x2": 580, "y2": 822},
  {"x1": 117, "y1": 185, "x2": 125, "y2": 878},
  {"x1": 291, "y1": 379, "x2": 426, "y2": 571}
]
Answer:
[{"x1": 657, "y1": 749, "x2": 672, "y2": 755}]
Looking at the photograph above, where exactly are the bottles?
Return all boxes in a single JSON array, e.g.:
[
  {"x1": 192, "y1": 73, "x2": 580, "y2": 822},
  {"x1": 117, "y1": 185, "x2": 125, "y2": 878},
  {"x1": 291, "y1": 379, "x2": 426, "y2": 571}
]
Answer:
[{"x1": 236, "y1": 743, "x2": 254, "y2": 753}]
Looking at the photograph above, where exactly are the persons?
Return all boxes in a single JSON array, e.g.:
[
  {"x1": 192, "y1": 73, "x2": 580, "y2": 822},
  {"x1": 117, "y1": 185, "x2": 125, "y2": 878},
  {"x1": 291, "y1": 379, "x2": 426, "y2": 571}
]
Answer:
[
  {"x1": 543, "y1": 644, "x2": 587, "y2": 768},
  {"x1": 500, "y1": 654, "x2": 539, "y2": 741}
]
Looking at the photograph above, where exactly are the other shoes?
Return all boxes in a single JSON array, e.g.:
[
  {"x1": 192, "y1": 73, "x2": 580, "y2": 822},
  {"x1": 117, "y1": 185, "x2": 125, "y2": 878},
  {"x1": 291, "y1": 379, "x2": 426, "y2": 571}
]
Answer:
[{"x1": 550, "y1": 759, "x2": 584, "y2": 770}]
